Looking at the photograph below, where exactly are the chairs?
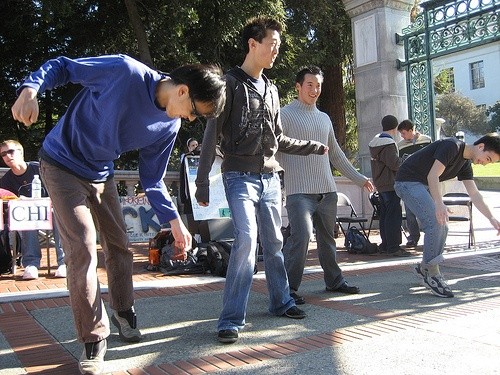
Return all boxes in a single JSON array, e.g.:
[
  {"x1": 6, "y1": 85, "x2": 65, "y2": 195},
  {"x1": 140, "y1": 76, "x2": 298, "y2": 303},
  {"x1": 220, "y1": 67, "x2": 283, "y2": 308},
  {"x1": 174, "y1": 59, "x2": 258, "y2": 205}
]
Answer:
[
  {"x1": 443, "y1": 192, "x2": 475, "y2": 248},
  {"x1": 335, "y1": 192, "x2": 371, "y2": 248},
  {"x1": 366, "y1": 191, "x2": 410, "y2": 245}
]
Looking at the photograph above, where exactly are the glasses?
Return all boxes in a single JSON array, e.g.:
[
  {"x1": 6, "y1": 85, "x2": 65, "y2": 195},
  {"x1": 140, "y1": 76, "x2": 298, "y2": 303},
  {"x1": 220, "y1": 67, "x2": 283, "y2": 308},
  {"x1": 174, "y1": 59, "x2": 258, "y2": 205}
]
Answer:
[
  {"x1": 190, "y1": 98, "x2": 201, "y2": 117},
  {"x1": 0, "y1": 148, "x2": 20, "y2": 157}
]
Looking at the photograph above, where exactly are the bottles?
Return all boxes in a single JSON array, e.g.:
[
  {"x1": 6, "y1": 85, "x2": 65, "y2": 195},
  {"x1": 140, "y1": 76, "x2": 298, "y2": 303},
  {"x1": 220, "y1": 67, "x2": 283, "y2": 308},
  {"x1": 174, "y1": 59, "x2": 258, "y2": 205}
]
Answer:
[
  {"x1": 149, "y1": 242, "x2": 159, "y2": 265},
  {"x1": 32, "y1": 174, "x2": 41, "y2": 199}
]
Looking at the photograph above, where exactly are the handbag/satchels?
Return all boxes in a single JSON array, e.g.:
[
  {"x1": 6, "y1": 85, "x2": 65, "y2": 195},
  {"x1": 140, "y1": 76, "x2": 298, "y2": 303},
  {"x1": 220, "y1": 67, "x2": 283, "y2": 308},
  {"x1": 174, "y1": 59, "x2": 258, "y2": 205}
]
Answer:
[{"x1": 143, "y1": 230, "x2": 208, "y2": 276}]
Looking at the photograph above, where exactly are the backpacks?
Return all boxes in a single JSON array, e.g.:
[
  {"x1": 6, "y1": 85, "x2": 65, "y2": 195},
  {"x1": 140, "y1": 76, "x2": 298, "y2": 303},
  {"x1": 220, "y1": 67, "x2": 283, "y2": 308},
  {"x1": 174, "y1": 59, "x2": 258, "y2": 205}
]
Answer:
[
  {"x1": 196, "y1": 239, "x2": 258, "y2": 278},
  {"x1": 344, "y1": 226, "x2": 368, "y2": 254}
]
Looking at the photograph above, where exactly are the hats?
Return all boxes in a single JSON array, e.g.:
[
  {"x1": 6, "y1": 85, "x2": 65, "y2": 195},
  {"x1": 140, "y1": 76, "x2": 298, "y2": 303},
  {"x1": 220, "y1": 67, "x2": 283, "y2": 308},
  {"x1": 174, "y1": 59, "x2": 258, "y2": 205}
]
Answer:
[{"x1": 381, "y1": 115, "x2": 398, "y2": 131}]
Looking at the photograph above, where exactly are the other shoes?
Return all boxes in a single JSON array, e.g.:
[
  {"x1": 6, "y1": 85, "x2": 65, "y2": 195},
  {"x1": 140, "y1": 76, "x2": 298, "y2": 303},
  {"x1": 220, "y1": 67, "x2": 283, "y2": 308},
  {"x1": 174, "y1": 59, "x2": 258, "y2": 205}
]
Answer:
[
  {"x1": 406, "y1": 239, "x2": 417, "y2": 247},
  {"x1": 378, "y1": 243, "x2": 411, "y2": 257}
]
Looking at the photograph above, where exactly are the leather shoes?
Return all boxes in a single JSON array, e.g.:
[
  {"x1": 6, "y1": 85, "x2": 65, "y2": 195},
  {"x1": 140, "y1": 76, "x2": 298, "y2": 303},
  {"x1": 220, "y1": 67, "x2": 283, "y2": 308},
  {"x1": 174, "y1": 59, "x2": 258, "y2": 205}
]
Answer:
[
  {"x1": 290, "y1": 292, "x2": 305, "y2": 305},
  {"x1": 326, "y1": 280, "x2": 360, "y2": 293}
]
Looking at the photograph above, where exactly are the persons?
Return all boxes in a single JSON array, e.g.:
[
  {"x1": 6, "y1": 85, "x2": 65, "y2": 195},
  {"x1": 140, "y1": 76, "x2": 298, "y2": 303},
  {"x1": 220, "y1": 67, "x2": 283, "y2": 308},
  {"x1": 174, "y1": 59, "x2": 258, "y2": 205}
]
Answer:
[
  {"x1": 194, "y1": 16, "x2": 330, "y2": 343},
  {"x1": 370, "y1": 115, "x2": 410, "y2": 257},
  {"x1": 181, "y1": 138, "x2": 198, "y2": 163},
  {"x1": 275, "y1": 66, "x2": 375, "y2": 303},
  {"x1": 11, "y1": 54, "x2": 226, "y2": 375},
  {"x1": 0, "y1": 140, "x2": 67, "y2": 280},
  {"x1": 394, "y1": 132, "x2": 500, "y2": 297},
  {"x1": 397, "y1": 120, "x2": 446, "y2": 248}
]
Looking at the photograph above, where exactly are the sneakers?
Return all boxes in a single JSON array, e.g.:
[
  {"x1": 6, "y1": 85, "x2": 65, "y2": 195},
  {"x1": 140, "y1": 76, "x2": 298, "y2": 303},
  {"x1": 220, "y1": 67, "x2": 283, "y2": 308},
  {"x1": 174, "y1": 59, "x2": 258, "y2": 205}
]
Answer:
[
  {"x1": 284, "y1": 306, "x2": 307, "y2": 319},
  {"x1": 414, "y1": 264, "x2": 454, "y2": 298},
  {"x1": 78, "y1": 338, "x2": 107, "y2": 375},
  {"x1": 54, "y1": 263, "x2": 67, "y2": 277},
  {"x1": 217, "y1": 329, "x2": 238, "y2": 343},
  {"x1": 111, "y1": 311, "x2": 142, "y2": 343},
  {"x1": 22, "y1": 265, "x2": 38, "y2": 280}
]
